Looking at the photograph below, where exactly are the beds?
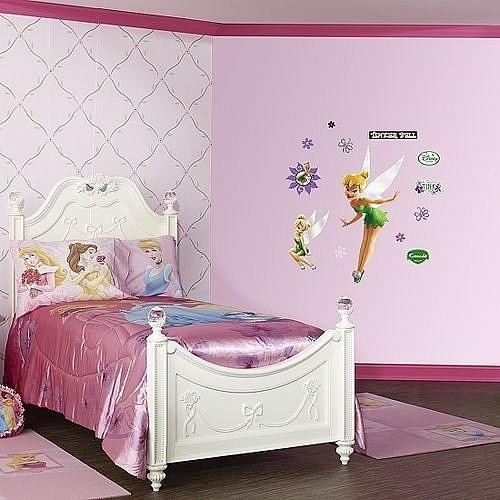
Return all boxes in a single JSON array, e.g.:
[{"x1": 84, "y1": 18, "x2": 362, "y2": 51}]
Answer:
[{"x1": 6, "y1": 173, "x2": 355, "y2": 493}]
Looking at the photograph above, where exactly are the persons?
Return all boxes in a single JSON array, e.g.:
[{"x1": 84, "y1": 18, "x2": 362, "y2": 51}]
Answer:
[
  {"x1": 131, "y1": 240, "x2": 182, "y2": 296},
  {"x1": 338, "y1": 170, "x2": 400, "y2": 284},
  {"x1": 56, "y1": 242, "x2": 119, "y2": 298},
  {"x1": 18, "y1": 246, "x2": 68, "y2": 305},
  {"x1": 289, "y1": 214, "x2": 317, "y2": 271},
  {"x1": 0, "y1": 391, "x2": 16, "y2": 431},
  {"x1": 8, "y1": 453, "x2": 47, "y2": 474}
]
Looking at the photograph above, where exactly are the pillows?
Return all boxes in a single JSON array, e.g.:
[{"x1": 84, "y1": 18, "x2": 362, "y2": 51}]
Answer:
[
  {"x1": 0, "y1": 386, "x2": 26, "y2": 437},
  {"x1": 9, "y1": 235, "x2": 183, "y2": 318}
]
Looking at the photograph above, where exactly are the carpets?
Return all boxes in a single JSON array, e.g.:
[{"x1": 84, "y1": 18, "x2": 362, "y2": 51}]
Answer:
[
  {"x1": 356, "y1": 393, "x2": 500, "y2": 460},
  {"x1": 0, "y1": 430, "x2": 133, "y2": 498}
]
